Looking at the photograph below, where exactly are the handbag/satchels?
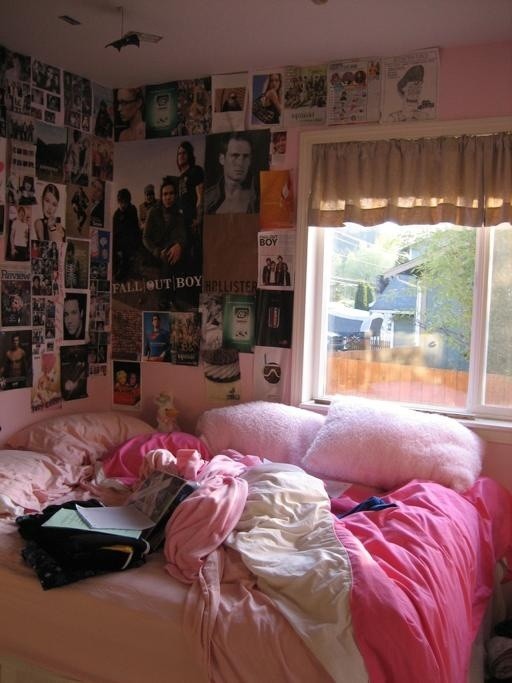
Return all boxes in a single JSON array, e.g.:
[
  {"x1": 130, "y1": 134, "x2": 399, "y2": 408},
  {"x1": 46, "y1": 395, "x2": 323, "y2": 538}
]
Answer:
[{"x1": 253, "y1": 94, "x2": 278, "y2": 123}]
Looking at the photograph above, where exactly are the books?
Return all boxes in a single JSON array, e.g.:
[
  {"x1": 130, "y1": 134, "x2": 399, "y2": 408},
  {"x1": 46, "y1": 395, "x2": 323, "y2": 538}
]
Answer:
[{"x1": 74, "y1": 469, "x2": 187, "y2": 529}]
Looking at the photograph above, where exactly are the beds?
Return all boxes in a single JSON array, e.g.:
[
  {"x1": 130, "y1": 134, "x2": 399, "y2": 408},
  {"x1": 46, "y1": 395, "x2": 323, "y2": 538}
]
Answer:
[{"x1": 0, "y1": 393, "x2": 511, "y2": 682}]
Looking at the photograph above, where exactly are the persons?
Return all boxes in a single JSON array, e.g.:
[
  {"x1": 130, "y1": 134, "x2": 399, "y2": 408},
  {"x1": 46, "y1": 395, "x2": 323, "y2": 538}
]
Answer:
[{"x1": 2, "y1": 51, "x2": 326, "y2": 411}]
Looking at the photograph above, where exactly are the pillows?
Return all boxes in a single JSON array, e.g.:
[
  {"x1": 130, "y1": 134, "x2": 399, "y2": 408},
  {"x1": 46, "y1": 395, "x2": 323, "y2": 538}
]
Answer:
[
  {"x1": 301, "y1": 396, "x2": 487, "y2": 493},
  {"x1": 0, "y1": 445, "x2": 81, "y2": 525},
  {"x1": 198, "y1": 399, "x2": 328, "y2": 468},
  {"x1": 1, "y1": 410, "x2": 161, "y2": 468}
]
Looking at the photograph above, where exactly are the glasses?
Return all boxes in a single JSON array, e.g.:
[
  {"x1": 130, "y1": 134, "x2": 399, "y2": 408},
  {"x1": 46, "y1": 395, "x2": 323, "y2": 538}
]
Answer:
[{"x1": 115, "y1": 98, "x2": 137, "y2": 106}]
